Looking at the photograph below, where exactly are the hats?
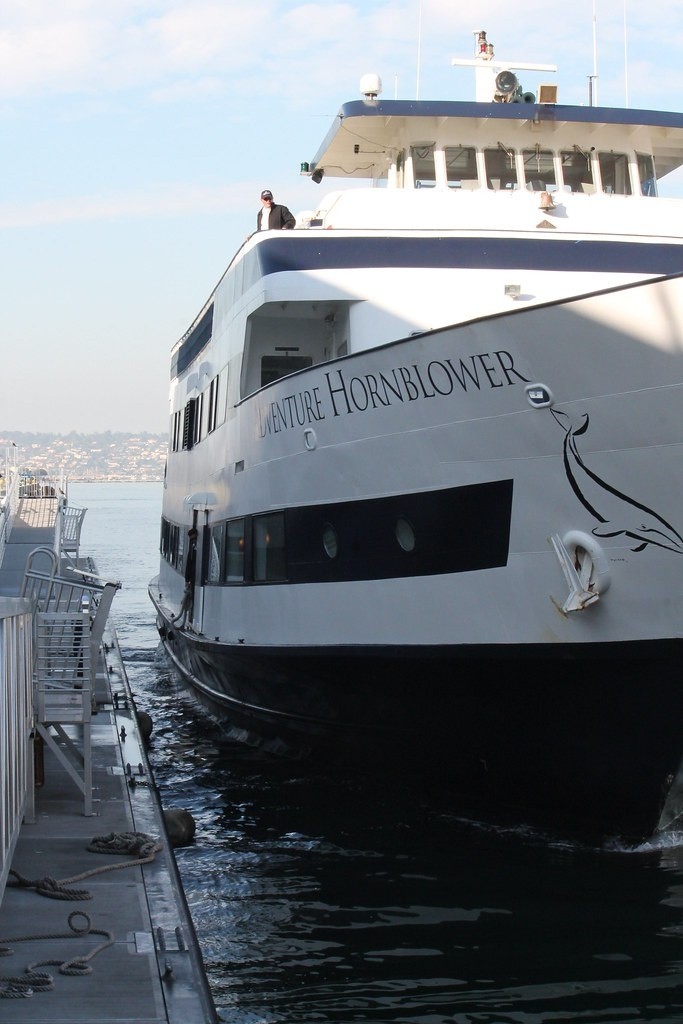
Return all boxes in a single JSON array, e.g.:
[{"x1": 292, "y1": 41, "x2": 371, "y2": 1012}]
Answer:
[{"x1": 261, "y1": 190, "x2": 273, "y2": 200}]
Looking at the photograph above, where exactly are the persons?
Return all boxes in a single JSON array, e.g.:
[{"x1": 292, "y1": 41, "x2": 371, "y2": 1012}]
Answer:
[{"x1": 245, "y1": 190, "x2": 296, "y2": 241}]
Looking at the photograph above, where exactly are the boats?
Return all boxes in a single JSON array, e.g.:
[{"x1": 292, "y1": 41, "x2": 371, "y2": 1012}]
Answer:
[{"x1": 147, "y1": 29, "x2": 683, "y2": 848}]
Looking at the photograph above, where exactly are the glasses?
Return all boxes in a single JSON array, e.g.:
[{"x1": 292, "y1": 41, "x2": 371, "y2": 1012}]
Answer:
[{"x1": 262, "y1": 198, "x2": 271, "y2": 201}]
